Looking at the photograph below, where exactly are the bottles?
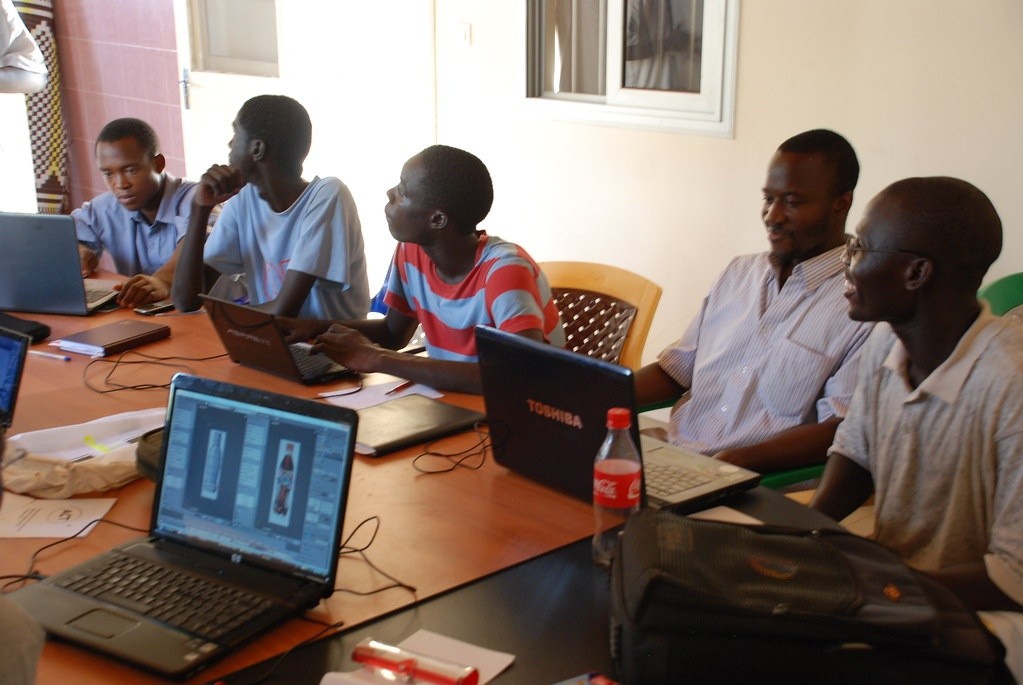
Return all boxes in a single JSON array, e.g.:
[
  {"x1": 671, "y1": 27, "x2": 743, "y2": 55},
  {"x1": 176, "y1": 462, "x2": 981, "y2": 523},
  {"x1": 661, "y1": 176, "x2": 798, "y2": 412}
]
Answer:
[
  {"x1": 593, "y1": 408, "x2": 642, "y2": 566},
  {"x1": 204, "y1": 433, "x2": 222, "y2": 491},
  {"x1": 273, "y1": 444, "x2": 295, "y2": 515}
]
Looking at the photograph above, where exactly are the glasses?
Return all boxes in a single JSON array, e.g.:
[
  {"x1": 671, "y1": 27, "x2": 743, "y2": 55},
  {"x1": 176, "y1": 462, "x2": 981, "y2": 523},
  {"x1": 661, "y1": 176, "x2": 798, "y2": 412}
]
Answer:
[{"x1": 847, "y1": 238, "x2": 937, "y2": 264}]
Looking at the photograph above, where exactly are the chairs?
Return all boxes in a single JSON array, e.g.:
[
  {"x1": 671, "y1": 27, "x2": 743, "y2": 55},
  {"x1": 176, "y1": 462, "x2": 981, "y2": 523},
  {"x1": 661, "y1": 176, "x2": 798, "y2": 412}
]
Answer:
[{"x1": 537, "y1": 261, "x2": 662, "y2": 372}]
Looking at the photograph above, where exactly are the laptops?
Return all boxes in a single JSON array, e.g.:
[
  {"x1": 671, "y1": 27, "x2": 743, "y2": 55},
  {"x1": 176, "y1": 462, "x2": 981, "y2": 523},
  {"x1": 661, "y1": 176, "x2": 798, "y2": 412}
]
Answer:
[
  {"x1": 0, "y1": 212, "x2": 125, "y2": 315},
  {"x1": 5, "y1": 372, "x2": 359, "y2": 678},
  {"x1": 198, "y1": 294, "x2": 358, "y2": 386},
  {"x1": 472, "y1": 322, "x2": 761, "y2": 514},
  {"x1": 0, "y1": 326, "x2": 30, "y2": 435}
]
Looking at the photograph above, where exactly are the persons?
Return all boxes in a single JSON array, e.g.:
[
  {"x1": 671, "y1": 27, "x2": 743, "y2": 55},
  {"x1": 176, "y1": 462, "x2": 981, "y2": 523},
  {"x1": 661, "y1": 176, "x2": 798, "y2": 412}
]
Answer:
[
  {"x1": 806, "y1": 176, "x2": 1023, "y2": 685},
  {"x1": 170, "y1": 94, "x2": 371, "y2": 320},
  {"x1": 625, "y1": 0, "x2": 690, "y2": 90},
  {"x1": 274, "y1": 144, "x2": 565, "y2": 394},
  {"x1": 633, "y1": 129, "x2": 875, "y2": 474},
  {"x1": 72, "y1": 118, "x2": 224, "y2": 308}
]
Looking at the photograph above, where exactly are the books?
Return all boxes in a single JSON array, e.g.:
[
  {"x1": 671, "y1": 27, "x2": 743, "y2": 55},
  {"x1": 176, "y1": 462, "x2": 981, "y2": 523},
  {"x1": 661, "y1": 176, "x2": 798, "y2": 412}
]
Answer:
[
  {"x1": 58, "y1": 318, "x2": 170, "y2": 356},
  {"x1": 355, "y1": 392, "x2": 486, "y2": 457}
]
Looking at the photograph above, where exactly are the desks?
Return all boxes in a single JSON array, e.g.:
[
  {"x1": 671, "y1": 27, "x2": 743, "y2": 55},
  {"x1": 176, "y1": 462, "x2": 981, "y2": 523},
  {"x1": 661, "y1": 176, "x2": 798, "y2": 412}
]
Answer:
[{"x1": 0, "y1": 270, "x2": 850, "y2": 685}]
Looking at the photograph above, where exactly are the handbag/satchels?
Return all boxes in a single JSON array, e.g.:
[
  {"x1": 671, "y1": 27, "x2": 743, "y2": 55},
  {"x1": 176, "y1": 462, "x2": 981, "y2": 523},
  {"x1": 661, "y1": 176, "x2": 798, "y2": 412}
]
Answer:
[{"x1": 611, "y1": 506, "x2": 1008, "y2": 683}]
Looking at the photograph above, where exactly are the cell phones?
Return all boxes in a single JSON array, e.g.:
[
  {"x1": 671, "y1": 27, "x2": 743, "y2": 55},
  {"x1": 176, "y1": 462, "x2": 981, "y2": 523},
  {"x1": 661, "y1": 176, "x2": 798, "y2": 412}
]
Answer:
[{"x1": 133, "y1": 300, "x2": 173, "y2": 316}]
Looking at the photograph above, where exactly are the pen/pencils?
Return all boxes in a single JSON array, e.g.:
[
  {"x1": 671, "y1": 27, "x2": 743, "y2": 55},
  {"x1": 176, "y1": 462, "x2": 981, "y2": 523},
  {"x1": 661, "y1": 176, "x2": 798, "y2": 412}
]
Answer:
[
  {"x1": 384, "y1": 380, "x2": 415, "y2": 396},
  {"x1": 27, "y1": 349, "x2": 72, "y2": 362}
]
